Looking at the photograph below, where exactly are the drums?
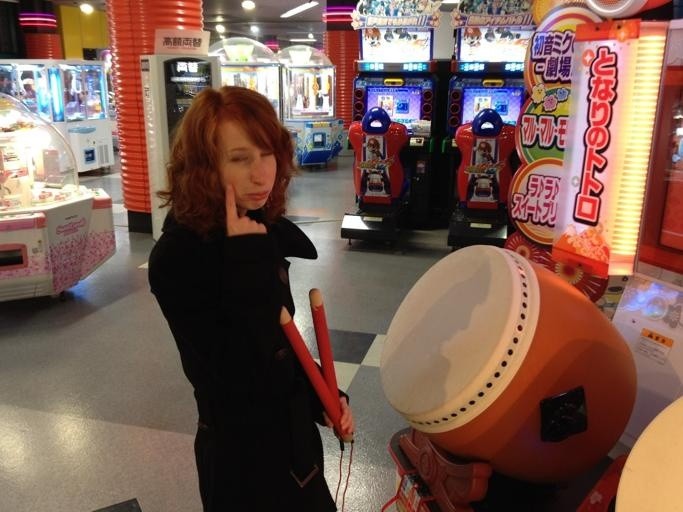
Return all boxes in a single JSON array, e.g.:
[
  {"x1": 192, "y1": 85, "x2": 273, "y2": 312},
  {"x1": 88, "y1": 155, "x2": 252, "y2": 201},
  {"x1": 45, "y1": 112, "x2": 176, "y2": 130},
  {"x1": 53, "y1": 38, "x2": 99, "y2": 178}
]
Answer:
[
  {"x1": 380, "y1": 245, "x2": 638, "y2": 485},
  {"x1": 615, "y1": 396, "x2": 683, "y2": 512}
]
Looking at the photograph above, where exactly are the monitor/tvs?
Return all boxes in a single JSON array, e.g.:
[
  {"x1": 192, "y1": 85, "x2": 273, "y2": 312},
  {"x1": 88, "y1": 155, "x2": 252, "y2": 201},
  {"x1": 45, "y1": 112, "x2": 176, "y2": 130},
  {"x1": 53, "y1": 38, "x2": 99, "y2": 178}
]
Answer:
[
  {"x1": 456, "y1": 82, "x2": 525, "y2": 131},
  {"x1": 362, "y1": 82, "x2": 422, "y2": 131}
]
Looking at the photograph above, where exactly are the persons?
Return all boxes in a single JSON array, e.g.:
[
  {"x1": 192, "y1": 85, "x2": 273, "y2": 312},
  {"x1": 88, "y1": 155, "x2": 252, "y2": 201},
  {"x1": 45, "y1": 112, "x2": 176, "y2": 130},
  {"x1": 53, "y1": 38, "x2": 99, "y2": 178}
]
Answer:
[
  {"x1": 366, "y1": 138, "x2": 384, "y2": 163},
  {"x1": 365, "y1": 27, "x2": 382, "y2": 50},
  {"x1": 476, "y1": 141, "x2": 494, "y2": 164},
  {"x1": 147, "y1": 85, "x2": 355, "y2": 512},
  {"x1": 463, "y1": 25, "x2": 482, "y2": 48}
]
